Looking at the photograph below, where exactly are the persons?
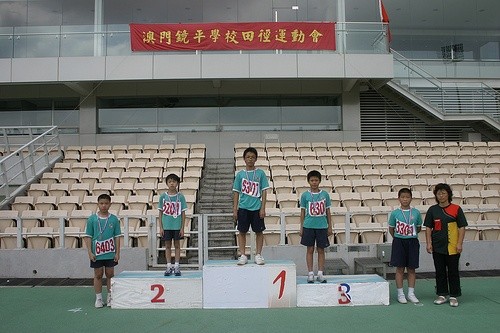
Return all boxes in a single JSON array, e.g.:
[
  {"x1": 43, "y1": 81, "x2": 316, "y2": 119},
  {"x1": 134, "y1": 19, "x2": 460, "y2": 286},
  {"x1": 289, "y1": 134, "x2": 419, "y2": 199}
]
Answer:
[
  {"x1": 232, "y1": 147, "x2": 270, "y2": 265},
  {"x1": 85, "y1": 194, "x2": 123, "y2": 308},
  {"x1": 423, "y1": 183, "x2": 468, "y2": 307},
  {"x1": 299, "y1": 170, "x2": 332, "y2": 283},
  {"x1": 157, "y1": 174, "x2": 187, "y2": 275},
  {"x1": 389, "y1": 188, "x2": 423, "y2": 304}
]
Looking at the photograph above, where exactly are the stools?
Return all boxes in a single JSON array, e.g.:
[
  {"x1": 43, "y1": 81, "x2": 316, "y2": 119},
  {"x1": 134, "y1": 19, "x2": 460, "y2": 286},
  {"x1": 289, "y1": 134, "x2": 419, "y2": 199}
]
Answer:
[
  {"x1": 354, "y1": 258, "x2": 386, "y2": 280},
  {"x1": 323, "y1": 258, "x2": 351, "y2": 275}
]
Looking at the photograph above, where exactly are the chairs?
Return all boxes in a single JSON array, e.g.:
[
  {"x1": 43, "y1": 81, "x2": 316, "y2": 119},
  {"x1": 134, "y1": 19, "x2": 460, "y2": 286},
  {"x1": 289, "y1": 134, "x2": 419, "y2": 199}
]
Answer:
[
  {"x1": 0, "y1": 144, "x2": 207, "y2": 266},
  {"x1": 233, "y1": 142, "x2": 500, "y2": 259}
]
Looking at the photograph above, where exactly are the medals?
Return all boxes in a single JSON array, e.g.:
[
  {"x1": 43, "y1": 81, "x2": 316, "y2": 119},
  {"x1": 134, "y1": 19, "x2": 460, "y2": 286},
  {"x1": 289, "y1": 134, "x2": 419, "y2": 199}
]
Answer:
[
  {"x1": 407, "y1": 229, "x2": 411, "y2": 234},
  {"x1": 249, "y1": 192, "x2": 252, "y2": 196},
  {"x1": 99, "y1": 233, "x2": 103, "y2": 238},
  {"x1": 313, "y1": 211, "x2": 317, "y2": 215},
  {"x1": 173, "y1": 213, "x2": 177, "y2": 218}
]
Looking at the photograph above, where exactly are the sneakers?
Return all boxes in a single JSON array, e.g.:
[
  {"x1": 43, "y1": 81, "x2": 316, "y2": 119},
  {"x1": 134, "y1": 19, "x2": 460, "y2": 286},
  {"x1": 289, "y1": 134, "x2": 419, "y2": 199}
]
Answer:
[
  {"x1": 317, "y1": 274, "x2": 327, "y2": 283},
  {"x1": 254, "y1": 254, "x2": 264, "y2": 264},
  {"x1": 407, "y1": 294, "x2": 420, "y2": 303},
  {"x1": 107, "y1": 296, "x2": 111, "y2": 306},
  {"x1": 307, "y1": 274, "x2": 314, "y2": 283},
  {"x1": 174, "y1": 265, "x2": 180, "y2": 276},
  {"x1": 237, "y1": 255, "x2": 247, "y2": 265},
  {"x1": 164, "y1": 267, "x2": 172, "y2": 275},
  {"x1": 397, "y1": 294, "x2": 407, "y2": 304},
  {"x1": 95, "y1": 299, "x2": 104, "y2": 308}
]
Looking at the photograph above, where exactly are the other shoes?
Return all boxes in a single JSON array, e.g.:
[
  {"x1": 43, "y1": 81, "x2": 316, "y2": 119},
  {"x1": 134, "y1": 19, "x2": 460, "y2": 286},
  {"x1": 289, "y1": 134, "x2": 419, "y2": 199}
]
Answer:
[
  {"x1": 449, "y1": 297, "x2": 458, "y2": 307},
  {"x1": 434, "y1": 296, "x2": 447, "y2": 304}
]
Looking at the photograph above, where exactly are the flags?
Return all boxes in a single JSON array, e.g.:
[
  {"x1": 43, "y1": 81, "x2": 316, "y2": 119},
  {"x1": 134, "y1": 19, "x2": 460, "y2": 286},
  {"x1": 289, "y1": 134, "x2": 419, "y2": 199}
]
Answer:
[{"x1": 377, "y1": 0, "x2": 393, "y2": 43}]
